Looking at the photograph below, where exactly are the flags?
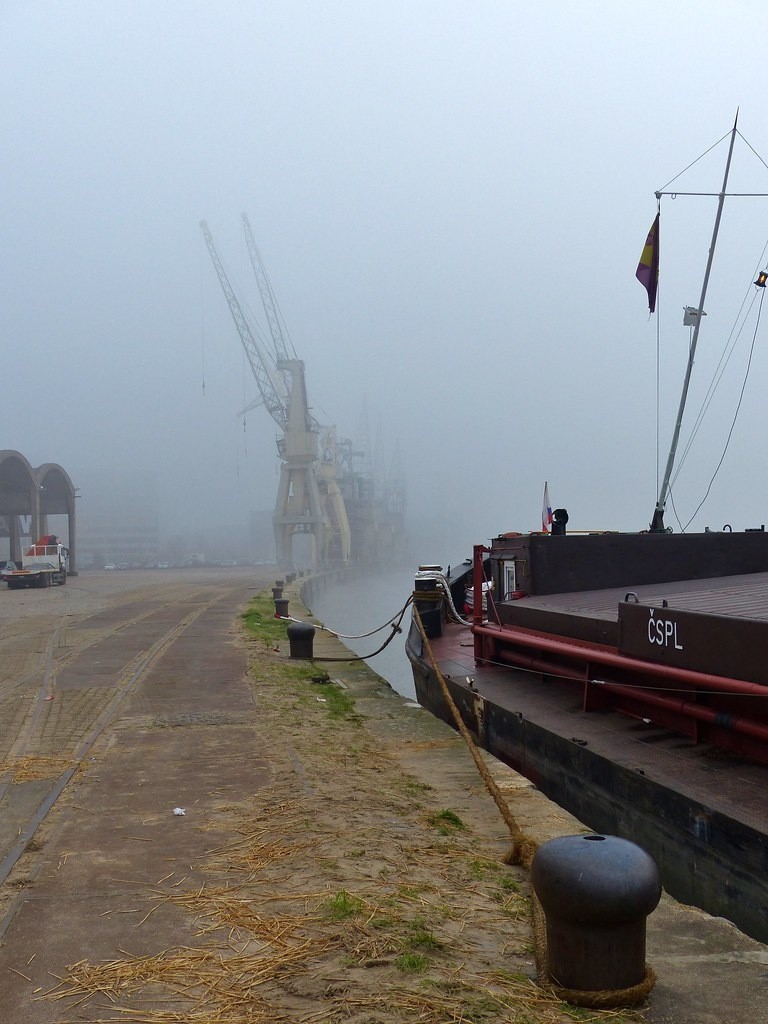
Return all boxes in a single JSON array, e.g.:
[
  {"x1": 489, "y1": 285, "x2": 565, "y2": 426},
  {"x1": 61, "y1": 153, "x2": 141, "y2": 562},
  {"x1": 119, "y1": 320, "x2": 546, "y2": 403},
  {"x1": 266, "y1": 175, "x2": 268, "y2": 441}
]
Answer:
[
  {"x1": 636, "y1": 213, "x2": 659, "y2": 313},
  {"x1": 542, "y1": 482, "x2": 553, "y2": 536}
]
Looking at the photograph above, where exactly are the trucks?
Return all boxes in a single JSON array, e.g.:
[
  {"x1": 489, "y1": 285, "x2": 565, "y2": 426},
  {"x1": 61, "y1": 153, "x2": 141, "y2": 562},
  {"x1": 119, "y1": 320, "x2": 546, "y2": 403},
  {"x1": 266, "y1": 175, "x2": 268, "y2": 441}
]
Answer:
[{"x1": 2, "y1": 544, "x2": 72, "y2": 588}]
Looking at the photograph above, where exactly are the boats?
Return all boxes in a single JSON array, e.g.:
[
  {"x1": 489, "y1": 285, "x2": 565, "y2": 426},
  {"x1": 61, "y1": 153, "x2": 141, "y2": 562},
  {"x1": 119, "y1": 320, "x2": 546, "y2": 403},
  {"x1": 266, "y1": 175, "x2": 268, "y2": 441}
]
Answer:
[{"x1": 404, "y1": 108, "x2": 768, "y2": 946}]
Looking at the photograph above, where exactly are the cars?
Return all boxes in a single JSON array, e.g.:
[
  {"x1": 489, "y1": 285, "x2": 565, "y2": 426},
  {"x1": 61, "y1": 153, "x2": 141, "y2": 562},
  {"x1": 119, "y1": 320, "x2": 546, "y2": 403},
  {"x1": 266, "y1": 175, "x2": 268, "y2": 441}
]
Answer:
[
  {"x1": 160, "y1": 563, "x2": 168, "y2": 569},
  {"x1": 105, "y1": 563, "x2": 116, "y2": 570},
  {"x1": 119, "y1": 563, "x2": 129, "y2": 570}
]
